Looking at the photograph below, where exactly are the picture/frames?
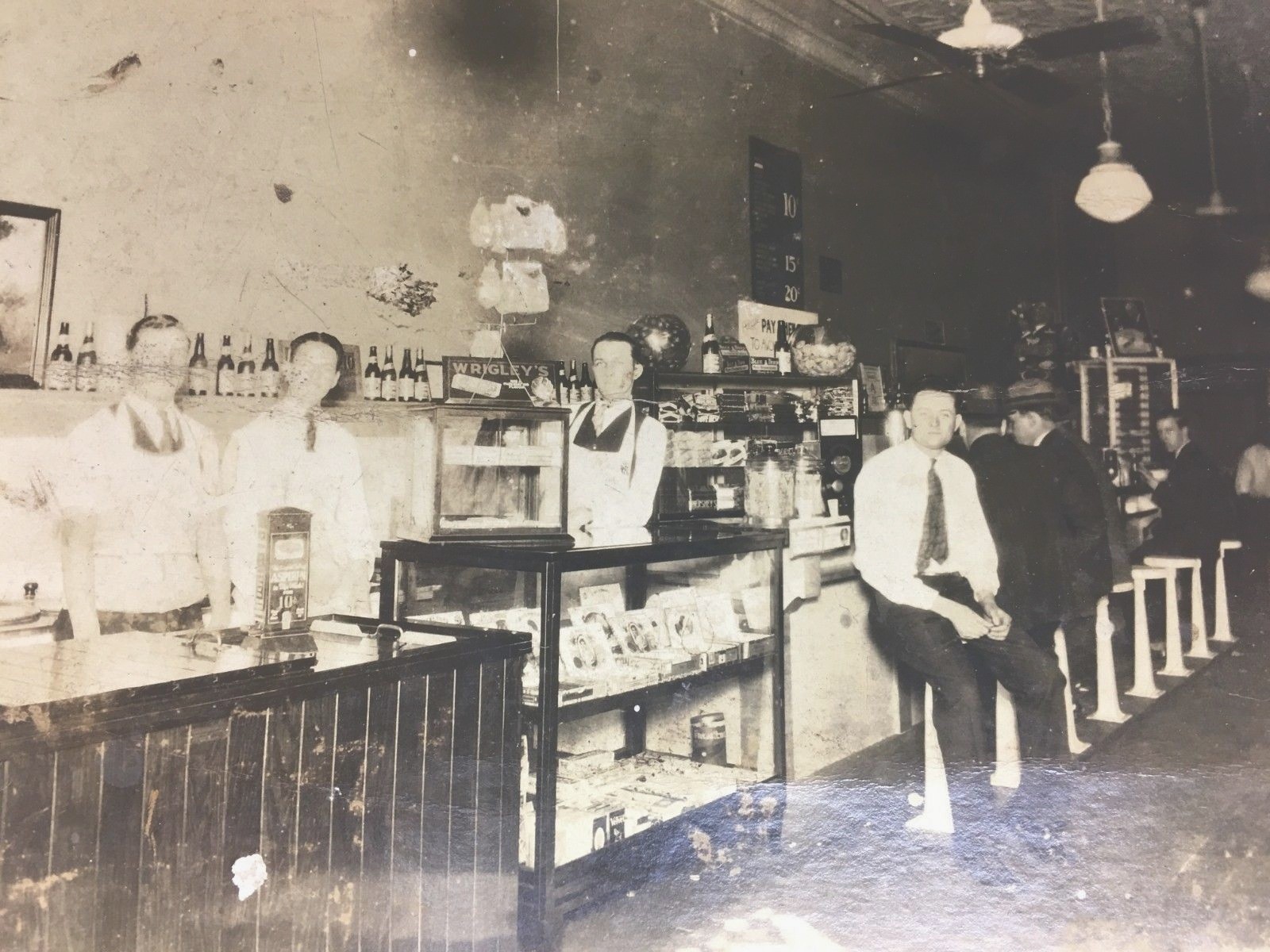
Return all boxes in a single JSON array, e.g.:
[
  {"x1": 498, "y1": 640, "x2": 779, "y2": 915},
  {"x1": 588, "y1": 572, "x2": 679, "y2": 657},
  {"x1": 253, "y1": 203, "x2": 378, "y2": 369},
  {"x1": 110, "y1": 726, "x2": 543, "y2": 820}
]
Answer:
[{"x1": 0, "y1": 200, "x2": 61, "y2": 389}]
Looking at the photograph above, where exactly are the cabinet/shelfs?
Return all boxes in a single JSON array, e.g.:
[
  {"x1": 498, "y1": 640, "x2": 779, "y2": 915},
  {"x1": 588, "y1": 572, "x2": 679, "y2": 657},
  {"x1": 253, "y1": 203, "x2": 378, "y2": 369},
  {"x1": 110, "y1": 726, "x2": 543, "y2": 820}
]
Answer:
[
  {"x1": 380, "y1": 521, "x2": 790, "y2": 952},
  {"x1": 633, "y1": 372, "x2": 861, "y2": 522}
]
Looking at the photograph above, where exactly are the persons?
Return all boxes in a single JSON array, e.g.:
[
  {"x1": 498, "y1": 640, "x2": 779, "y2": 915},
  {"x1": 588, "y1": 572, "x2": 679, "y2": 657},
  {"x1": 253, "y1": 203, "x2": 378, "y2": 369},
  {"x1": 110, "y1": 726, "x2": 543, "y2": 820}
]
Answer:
[
  {"x1": 220, "y1": 332, "x2": 384, "y2": 626},
  {"x1": 53, "y1": 315, "x2": 232, "y2": 640},
  {"x1": 566, "y1": 331, "x2": 669, "y2": 528},
  {"x1": 851, "y1": 378, "x2": 1270, "y2": 887}
]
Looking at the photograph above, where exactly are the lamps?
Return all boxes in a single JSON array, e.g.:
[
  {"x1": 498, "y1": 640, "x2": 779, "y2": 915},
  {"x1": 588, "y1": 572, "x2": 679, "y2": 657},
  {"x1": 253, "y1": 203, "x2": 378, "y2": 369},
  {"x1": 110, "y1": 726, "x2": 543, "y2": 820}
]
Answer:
[
  {"x1": 810, "y1": 0, "x2": 1163, "y2": 105},
  {"x1": 1074, "y1": 0, "x2": 1152, "y2": 221}
]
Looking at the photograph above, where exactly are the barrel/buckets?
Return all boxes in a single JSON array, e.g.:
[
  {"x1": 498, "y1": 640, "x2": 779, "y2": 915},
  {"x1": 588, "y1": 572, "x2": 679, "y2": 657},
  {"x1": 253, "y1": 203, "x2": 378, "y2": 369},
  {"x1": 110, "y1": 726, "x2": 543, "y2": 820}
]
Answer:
[{"x1": 690, "y1": 712, "x2": 727, "y2": 767}]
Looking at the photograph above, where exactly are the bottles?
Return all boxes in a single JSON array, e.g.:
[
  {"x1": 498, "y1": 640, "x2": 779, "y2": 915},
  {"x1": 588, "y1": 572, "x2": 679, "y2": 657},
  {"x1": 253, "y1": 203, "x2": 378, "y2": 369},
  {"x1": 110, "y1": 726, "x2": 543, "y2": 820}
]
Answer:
[
  {"x1": 398, "y1": 347, "x2": 430, "y2": 403},
  {"x1": 774, "y1": 320, "x2": 791, "y2": 377},
  {"x1": 48, "y1": 321, "x2": 97, "y2": 392},
  {"x1": 702, "y1": 314, "x2": 720, "y2": 373},
  {"x1": 863, "y1": 384, "x2": 869, "y2": 415},
  {"x1": 559, "y1": 359, "x2": 603, "y2": 405},
  {"x1": 364, "y1": 345, "x2": 397, "y2": 402},
  {"x1": 1129, "y1": 454, "x2": 1141, "y2": 492},
  {"x1": 215, "y1": 334, "x2": 280, "y2": 398},
  {"x1": 188, "y1": 333, "x2": 208, "y2": 396},
  {"x1": 744, "y1": 444, "x2": 826, "y2": 530}
]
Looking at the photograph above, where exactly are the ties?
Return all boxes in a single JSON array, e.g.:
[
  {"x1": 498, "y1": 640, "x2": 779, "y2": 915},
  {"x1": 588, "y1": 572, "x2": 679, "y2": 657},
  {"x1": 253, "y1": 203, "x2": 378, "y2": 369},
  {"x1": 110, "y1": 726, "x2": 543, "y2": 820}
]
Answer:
[
  {"x1": 157, "y1": 410, "x2": 173, "y2": 453},
  {"x1": 304, "y1": 413, "x2": 317, "y2": 452},
  {"x1": 917, "y1": 458, "x2": 949, "y2": 574}
]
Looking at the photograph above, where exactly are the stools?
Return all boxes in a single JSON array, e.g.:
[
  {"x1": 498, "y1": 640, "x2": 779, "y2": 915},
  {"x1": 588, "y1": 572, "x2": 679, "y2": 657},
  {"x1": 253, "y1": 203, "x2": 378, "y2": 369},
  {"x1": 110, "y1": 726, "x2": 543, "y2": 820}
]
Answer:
[{"x1": 907, "y1": 540, "x2": 1242, "y2": 834}]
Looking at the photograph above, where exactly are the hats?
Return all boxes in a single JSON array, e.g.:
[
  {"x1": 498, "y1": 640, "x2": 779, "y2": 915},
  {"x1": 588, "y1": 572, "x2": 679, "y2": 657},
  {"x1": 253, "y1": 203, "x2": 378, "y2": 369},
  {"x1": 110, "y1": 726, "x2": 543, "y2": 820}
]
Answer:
[
  {"x1": 960, "y1": 382, "x2": 1006, "y2": 417},
  {"x1": 1002, "y1": 378, "x2": 1059, "y2": 410}
]
